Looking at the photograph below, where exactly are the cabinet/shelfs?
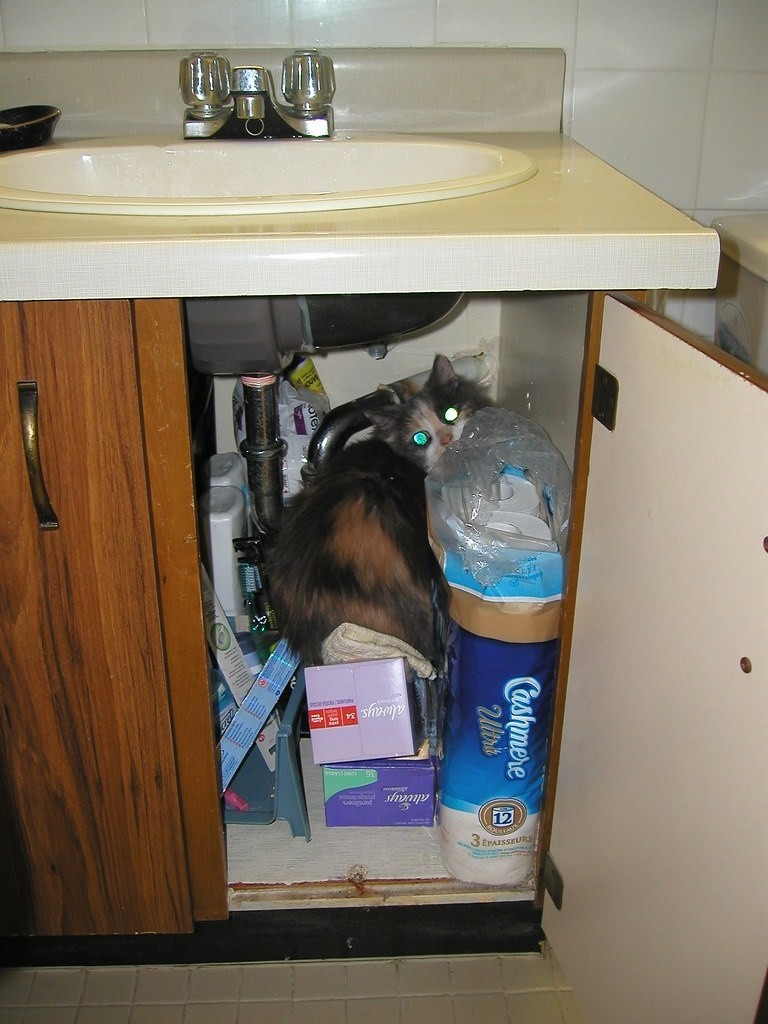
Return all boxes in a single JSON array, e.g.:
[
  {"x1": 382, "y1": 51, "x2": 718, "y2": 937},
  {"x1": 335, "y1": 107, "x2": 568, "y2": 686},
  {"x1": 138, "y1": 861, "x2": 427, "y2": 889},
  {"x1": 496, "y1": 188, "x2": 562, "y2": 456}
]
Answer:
[{"x1": 0, "y1": 290, "x2": 768, "y2": 1024}]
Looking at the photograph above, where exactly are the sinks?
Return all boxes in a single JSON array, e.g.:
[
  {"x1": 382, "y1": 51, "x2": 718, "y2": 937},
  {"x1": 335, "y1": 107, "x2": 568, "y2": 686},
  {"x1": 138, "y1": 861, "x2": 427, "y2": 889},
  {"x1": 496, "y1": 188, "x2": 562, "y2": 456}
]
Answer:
[{"x1": 0, "y1": 130, "x2": 534, "y2": 217}]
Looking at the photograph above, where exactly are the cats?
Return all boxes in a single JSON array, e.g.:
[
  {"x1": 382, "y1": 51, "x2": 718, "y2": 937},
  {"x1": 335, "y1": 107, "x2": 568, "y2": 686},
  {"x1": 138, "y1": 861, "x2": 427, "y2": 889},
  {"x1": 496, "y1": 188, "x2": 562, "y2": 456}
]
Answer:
[{"x1": 257, "y1": 353, "x2": 497, "y2": 665}]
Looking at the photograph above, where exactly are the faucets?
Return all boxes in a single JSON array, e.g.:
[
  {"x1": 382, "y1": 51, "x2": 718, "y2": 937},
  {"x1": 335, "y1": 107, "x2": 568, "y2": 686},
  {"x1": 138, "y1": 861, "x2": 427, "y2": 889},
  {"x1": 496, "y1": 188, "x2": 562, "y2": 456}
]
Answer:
[{"x1": 177, "y1": 47, "x2": 341, "y2": 140}]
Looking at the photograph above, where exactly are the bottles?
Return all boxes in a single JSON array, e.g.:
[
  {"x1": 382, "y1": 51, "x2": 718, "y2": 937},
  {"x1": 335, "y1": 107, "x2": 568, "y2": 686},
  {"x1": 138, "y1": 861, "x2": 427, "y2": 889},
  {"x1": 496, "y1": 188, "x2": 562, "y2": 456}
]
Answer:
[
  {"x1": 203, "y1": 451, "x2": 252, "y2": 539},
  {"x1": 197, "y1": 484, "x2": 246, "y2": 633}
]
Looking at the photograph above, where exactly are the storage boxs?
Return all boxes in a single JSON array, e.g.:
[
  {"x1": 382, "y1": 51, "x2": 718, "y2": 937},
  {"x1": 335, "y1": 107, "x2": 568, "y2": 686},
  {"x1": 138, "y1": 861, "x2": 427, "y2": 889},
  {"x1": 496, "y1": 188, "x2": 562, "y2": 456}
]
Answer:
[
  {"x1": 319, "y1": 759, "x2": 435, "y2": 828},
  {"x1": 304, "y1": 656, "x2": 422, "y2": 766}
]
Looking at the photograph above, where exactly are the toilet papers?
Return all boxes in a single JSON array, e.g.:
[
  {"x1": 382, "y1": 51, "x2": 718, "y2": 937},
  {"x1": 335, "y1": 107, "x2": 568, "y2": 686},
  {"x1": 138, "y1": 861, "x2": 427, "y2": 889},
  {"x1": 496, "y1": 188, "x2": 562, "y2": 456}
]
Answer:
[{"x1": 442, "y1": 474, "x2": 553, "y2": 615}]
